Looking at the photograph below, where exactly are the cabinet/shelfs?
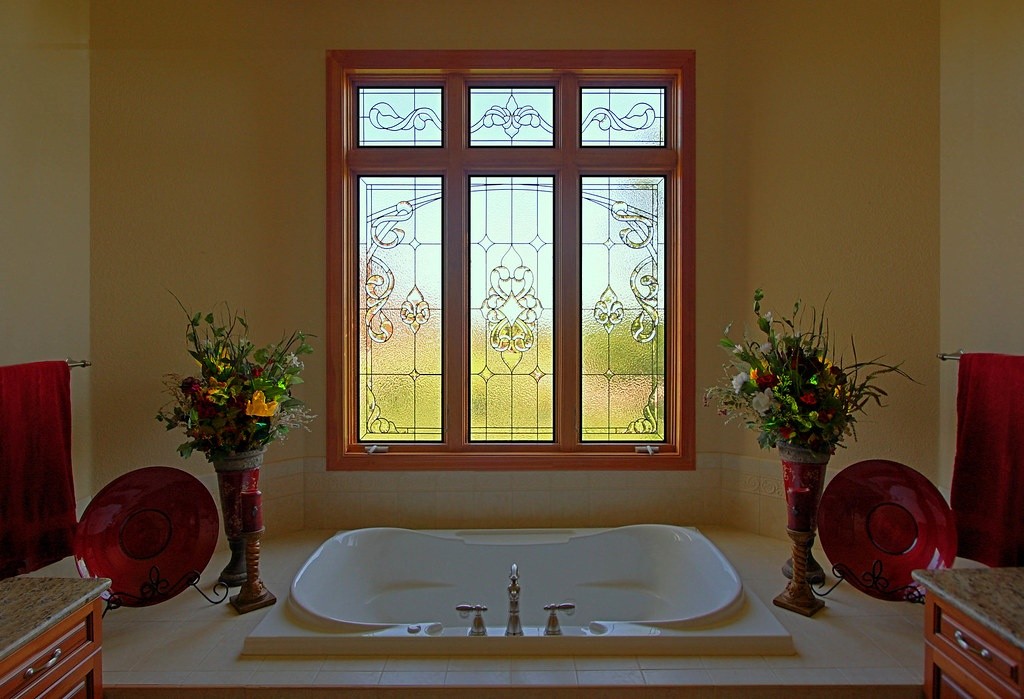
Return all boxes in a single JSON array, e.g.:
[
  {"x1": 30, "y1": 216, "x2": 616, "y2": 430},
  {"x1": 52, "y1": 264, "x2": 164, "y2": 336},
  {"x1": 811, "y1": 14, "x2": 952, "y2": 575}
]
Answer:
[
  {"x1": 0, "y1": 574, "x2": 112, "y2": 699},
  {"x1": 910, "y1": 566, "x2": 1024, "y2": 699}
]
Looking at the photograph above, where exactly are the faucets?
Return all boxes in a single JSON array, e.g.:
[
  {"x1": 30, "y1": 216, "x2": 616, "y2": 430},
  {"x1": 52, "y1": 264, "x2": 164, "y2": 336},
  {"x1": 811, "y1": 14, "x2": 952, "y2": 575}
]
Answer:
[{"x1": 504, "y1": 562, "x2": 526, "y2": 638}]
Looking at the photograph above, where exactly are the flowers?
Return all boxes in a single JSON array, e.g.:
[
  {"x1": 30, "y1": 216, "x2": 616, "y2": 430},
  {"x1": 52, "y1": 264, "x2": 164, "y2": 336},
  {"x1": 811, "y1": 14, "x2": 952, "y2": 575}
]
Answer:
[
  {"x1": 155, "y1": 284, "x2": 318, "y2": 464},
  {"x1": 704, "y1": 286, "x2": 926, "y2": 455}
]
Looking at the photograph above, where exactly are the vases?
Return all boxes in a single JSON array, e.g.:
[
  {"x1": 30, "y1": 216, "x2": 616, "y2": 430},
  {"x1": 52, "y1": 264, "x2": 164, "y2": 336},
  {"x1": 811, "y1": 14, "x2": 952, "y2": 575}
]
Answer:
[
  {"x1": 213, "y1": 447, "x2": 269, "y2": 587},
  {"x1": 778, "y1": 449, "x2": 832, "y2": 584}
]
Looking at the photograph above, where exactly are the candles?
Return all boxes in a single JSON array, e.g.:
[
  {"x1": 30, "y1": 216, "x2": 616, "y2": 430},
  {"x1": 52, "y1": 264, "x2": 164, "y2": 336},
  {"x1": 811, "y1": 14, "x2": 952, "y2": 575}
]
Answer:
[
  {"x1": 787, "y1": 488, "x2": 811, "y2": 532},
  {"x1": 241, "y1": 490, "x2": 263, "y2": 532}
]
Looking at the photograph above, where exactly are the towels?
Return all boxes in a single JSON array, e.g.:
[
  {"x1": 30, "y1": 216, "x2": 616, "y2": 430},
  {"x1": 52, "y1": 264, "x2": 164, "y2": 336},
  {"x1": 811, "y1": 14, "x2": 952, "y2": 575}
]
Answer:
[
  {"x1": 0, "y1": 361, "x2": 79, "y2": 581},
  {"x1": 950, "y1": 351, "x2": 1023, "y2": 567}
]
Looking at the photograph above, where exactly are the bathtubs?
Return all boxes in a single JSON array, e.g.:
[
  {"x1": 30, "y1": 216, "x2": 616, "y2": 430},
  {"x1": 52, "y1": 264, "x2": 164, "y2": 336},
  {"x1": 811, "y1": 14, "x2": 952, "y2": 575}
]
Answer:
[{"x1": 239, "y1": 522, "x2": 799, "y2": 657}]
